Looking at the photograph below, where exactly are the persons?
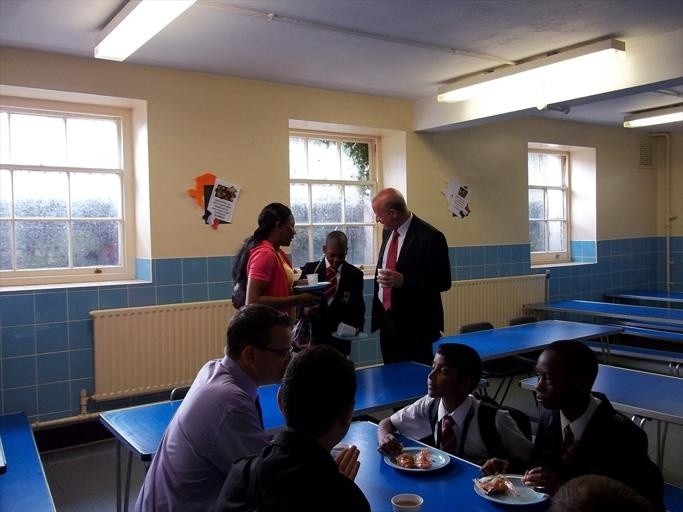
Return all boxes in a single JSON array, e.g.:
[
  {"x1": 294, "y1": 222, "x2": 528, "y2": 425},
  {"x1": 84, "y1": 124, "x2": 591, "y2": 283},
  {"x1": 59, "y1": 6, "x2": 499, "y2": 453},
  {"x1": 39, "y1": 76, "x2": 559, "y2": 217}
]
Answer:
[
  {"x1": 231, "y1": 201, "x2": 322, "y2": 332},
  {"x1": 295, "y1": 229, "x2": 365, "y2": 362},
  {"x1": 369, "y1": 187, "x2": 451, "y2": 367},
  {"x1": 212, "y1": 344, "x2": 372, "y2": 511},
  {"x1": 479, "y1": 340, "x2": 664, "y2": 511},
  {"x1": 546, "y1": 474, "x2": 657, "y2": 512},
  {"x1": 376, "y1": 342, "x2": 534, "y2": 470},
  {"x1": 131, "y1": 301, "x2": 292, "y2": 512}
]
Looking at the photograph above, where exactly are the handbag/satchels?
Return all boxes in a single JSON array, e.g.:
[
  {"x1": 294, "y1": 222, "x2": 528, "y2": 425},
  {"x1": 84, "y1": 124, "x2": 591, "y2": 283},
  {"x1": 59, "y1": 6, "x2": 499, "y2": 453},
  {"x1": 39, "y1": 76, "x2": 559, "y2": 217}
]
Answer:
[
  {"x1": 230, "y1": 279, "x2": 245, "y2": 309},
  {"x1": 290, "y1": 319, "x2": 311, "y2": 352}
]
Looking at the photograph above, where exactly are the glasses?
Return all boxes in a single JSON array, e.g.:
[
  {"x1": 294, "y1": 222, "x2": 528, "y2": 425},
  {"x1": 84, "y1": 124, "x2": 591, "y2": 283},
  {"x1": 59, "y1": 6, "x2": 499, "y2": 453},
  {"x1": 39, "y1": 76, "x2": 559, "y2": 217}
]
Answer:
[{"x1": 268, "y1": 346, "x2": 293, "y2": 357}]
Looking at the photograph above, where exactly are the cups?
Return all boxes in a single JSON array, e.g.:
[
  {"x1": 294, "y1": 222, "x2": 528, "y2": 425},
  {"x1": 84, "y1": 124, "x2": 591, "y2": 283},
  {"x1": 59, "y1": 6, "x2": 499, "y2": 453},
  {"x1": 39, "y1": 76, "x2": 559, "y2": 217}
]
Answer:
[
  {"x1": 336, "y1": 322, "x2": 357, "y2": 338},
  {"x1": 389, "y1": 493, "x2": 424, "y2": 512},
  {"x1": 377, "y1": 268, "x2": 391, "y2": 287},
  {"x1": 306, "y1": 273, "x2": 318, "y2": 287},
  {"x1": 328, "y1": 442, "x2": 354, "y2": 459}
]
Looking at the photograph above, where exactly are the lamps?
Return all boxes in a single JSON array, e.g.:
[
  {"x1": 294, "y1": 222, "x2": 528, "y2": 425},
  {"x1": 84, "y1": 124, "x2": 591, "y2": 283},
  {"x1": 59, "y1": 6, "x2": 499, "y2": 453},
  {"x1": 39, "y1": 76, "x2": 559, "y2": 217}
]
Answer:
[
  {"x1": 436, "y1": 39, "x2": 625, "y2": 104},
  {"x1": 623, "y1": 102, "x2": 683, "y2": 130},
  {"x1": 92, "y1": 0, "x2": 200, "y2": 65}
]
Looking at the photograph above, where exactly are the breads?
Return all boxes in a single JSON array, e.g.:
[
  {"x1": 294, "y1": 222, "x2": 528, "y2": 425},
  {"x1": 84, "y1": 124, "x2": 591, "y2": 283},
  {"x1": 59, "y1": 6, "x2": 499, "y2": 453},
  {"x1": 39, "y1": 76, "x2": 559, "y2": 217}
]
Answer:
[
  {"x1": 395, "y1": 453, "x2": 415, "y2": 468},
  {"x1": 480, "y1": 474, "x2": 508, "y2": 493},
  {"x1": 416, "y1": 452, "x2": 430, "y2": 468}
]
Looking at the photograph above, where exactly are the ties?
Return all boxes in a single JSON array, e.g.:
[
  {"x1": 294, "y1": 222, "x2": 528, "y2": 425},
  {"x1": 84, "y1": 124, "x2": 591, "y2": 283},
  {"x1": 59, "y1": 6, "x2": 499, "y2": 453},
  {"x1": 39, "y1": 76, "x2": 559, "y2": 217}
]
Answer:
[
  {"x1": 304, "y1": 267, "x2": 338, "y2": 316},
  {"x1": 442, "y1": 418, "x2": 456, "y2": 453},
  {"x1": 382, "y1": 230, "x2": 399, "y2": 311},
  {"x1": 561, "y1": 425, "x2": 576, "y2": 484}
]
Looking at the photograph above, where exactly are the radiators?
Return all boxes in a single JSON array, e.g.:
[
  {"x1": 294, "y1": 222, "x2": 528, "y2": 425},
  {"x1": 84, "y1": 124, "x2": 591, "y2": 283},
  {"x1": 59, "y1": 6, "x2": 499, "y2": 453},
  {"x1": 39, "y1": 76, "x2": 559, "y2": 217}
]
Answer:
[
  {"x1": 437, "y1": 270, "x2": 550, "y2": 336},
  {"x1": 89, "y1": 298, "x2": 240, "y2": 402}
]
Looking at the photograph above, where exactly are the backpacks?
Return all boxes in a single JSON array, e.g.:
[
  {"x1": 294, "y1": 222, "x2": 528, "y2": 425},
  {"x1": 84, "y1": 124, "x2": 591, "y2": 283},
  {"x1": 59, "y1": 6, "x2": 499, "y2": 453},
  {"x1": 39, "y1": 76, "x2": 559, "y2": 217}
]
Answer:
[
  {"x1": 545, "y1": 412, "x2": 665, "y2": 509},
  {"x1": 426, "y1": 392, "x2": 532, "y2": 460}
]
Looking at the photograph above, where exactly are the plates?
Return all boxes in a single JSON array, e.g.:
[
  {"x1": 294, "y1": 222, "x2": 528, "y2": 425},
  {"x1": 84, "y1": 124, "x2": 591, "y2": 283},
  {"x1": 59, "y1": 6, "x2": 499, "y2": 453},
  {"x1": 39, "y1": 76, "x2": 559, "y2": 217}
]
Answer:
[
  {"x1": 471, "y1": 473, "x2": 550, "y2": 506},
  {"x1": 382, "y1": 446, "x2": 452, "y2": 474}
]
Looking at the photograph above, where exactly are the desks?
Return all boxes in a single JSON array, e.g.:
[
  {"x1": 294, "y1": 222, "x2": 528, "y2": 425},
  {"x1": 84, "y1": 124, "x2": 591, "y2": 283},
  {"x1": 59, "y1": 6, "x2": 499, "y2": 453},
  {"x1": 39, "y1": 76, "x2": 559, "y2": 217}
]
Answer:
[
  {"x1": 519, "y1": 364, "x2": 682, "y2": 425},
  {"x1": 433, "y1": 292, "x2": 683, "y2": 376},
  {"x1": 99, "y1": 360, "x2": 490, "y2": 512},
  {"x1": 0, "y1": 410, "x2": 57, "y2": 512}
]
